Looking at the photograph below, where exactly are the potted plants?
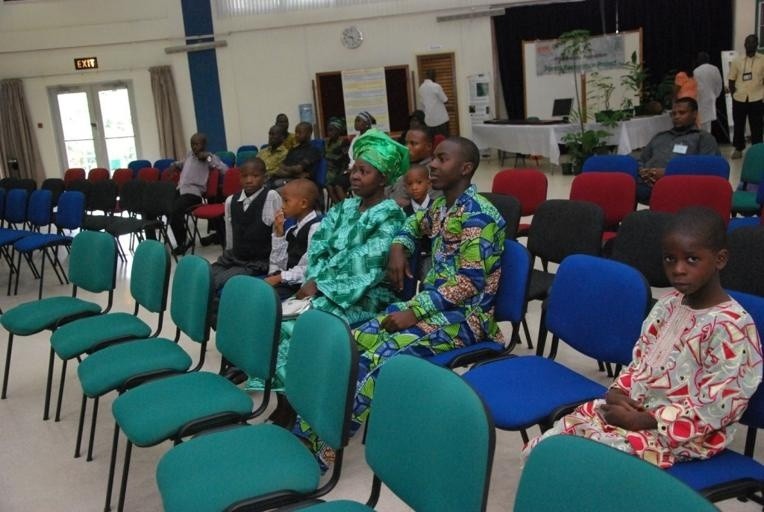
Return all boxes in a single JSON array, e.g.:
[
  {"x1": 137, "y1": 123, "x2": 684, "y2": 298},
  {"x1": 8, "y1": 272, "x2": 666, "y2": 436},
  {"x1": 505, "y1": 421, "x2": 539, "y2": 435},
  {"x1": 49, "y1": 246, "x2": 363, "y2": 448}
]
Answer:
[{"x1": 549, "y1": 30, "x2": 662, "y2": 174}]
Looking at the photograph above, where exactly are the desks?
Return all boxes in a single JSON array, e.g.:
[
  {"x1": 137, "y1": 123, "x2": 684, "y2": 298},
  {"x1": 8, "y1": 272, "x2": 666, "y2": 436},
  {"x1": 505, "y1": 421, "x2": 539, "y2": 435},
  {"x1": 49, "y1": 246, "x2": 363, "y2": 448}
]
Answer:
[{"x1": 472, "y1": 109, "x2": 674, "y2": 169}]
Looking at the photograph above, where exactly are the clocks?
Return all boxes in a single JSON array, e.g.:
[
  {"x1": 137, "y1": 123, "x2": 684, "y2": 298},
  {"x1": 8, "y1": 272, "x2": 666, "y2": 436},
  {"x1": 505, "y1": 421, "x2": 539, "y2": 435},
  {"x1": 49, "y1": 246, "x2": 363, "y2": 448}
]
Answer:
[{"x1": 341, "y1": 26, "x2": 362, "y2": 49}]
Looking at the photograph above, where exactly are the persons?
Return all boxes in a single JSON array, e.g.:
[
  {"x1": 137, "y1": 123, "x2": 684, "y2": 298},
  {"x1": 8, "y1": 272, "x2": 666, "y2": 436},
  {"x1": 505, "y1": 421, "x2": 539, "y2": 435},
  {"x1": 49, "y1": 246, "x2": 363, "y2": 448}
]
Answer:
[
  {"x1": 692, "y1": 51, "x2": 723, "y2": 133},
  {"x1": 161, "y1": 66, "x2": 505, "y2": 480},
  {"x1": 632, "y1": 97, "x2": 719, "y2": 204},
  {"x1": 727, "y1": 34, "x2": 764, "y2": 160},
  {"x1": 516, "y1": 205, "x2": 764, "y2": 476},
  {"x1": 674, "y1": 70, "x2": 701, "y2": 128}
]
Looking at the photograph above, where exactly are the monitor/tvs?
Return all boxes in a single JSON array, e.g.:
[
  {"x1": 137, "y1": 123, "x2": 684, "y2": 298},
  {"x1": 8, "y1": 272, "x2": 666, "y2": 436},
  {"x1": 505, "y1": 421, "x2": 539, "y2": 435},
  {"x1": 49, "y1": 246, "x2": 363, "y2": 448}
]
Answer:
[{"x1": 552, "y1": 98, "x2": 573, "y2": 123}]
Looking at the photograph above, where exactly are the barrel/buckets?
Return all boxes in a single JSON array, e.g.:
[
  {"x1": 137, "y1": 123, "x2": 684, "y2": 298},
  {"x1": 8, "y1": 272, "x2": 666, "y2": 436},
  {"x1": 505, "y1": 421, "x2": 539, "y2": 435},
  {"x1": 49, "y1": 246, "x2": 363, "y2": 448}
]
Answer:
[{"x1": 298, "y1": 104, "x2": 313, "y2": 124}]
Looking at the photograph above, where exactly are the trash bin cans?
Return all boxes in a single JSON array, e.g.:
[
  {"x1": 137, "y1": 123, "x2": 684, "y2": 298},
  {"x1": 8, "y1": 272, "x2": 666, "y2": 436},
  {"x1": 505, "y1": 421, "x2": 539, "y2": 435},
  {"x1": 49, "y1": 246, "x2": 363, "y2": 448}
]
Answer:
[{"x1": 7, "y1": 158, "x2": 19, "y2": 180}]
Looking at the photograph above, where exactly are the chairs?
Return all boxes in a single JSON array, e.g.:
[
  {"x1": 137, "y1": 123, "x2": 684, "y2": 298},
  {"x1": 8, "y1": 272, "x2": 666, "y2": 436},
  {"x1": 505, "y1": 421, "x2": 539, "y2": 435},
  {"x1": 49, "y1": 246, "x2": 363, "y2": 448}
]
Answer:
[{"x1": 0, "y1": 138, "x2": 764, "y2": 512}]
